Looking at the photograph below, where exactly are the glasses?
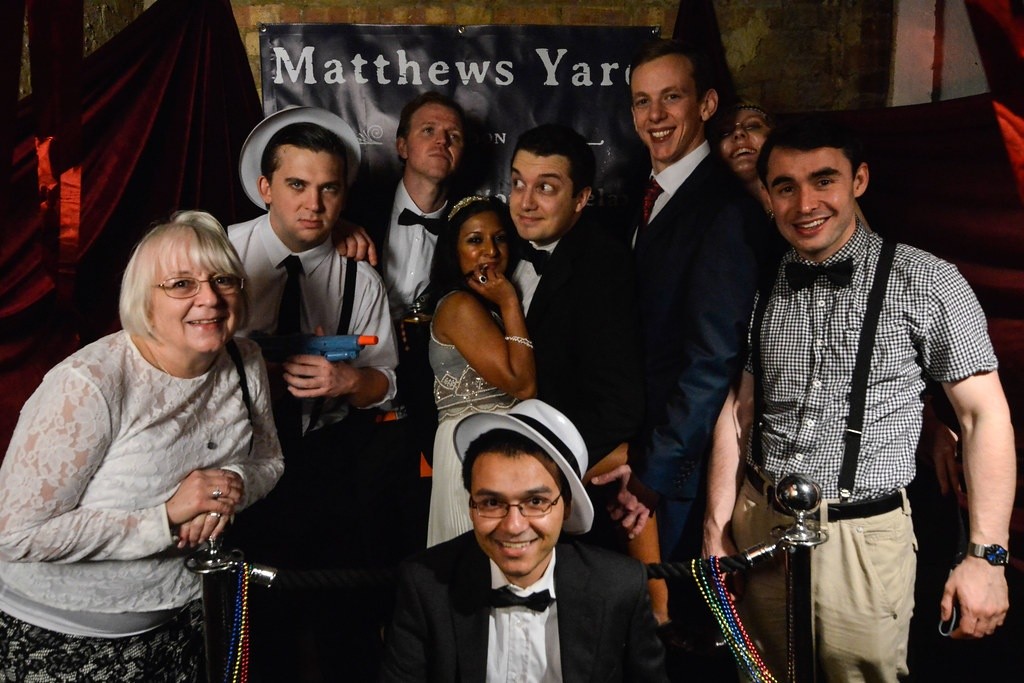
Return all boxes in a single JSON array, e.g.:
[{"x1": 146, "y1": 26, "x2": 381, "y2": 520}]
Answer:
[
  {"x1": 153, "y1": 272, "x2": 238, "y2": 299},
  {"x1": 463, "y1": 491, "x2": 562, "y2": 518}
]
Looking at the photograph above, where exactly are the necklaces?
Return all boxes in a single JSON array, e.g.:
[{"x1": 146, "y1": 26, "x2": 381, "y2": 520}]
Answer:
[
  {"x1": 691, "y1": 555, "x2": 778, "y2": 683},
  {"x1": 225, "y1": 562, "x2": 249, "y2": 683},
  {"x1": 156, "y1": 359, "x2": 217, "y2": 450}
]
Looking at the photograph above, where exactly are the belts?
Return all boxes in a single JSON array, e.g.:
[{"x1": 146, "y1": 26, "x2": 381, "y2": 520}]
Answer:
[{"x1": 746, "y1": 467, "x2": 904, "y2": 523}]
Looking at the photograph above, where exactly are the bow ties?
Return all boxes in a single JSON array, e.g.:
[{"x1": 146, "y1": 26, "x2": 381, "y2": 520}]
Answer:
[
  {"x1": 519, "y1": 244, "x2": 552, "y2": 276},
  {"x1": 782, "y1": 257, "x2": 861, "y2": 290},
  {"x1": 492, "y1": 589, "x2": 556, "y2": 613},
  {"x1": 395, "y1": 207, "x2": 445, "y2": 236}
]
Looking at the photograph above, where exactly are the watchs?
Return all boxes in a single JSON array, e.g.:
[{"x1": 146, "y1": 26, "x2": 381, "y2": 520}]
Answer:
[{"x1": 969, "y1": 542, "x2": 1008, "y2": 565}]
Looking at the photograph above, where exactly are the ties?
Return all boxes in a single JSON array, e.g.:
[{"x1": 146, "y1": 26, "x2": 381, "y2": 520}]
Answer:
[
  {"x1": 637, "y1": 176, "x2": 669, "y2": 224},
  {"x1": 275, "y1": 255, "x2": 306, "y2": 338}
]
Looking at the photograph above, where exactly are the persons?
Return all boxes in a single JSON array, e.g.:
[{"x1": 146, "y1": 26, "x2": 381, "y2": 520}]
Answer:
[
  {"x1": 227, "y1": 103, "x2": 400, "y2": 682},
  {"x1": 377, "y1": 398, "x2": 677, "y2": 683},
  {"x1": 507, "y1": 122, "x2": 636, "y2": 474},
  {"x1": 697, "y1": 119, "x2": 1016, "y2": 683},
  {"x1": 709, "y1": 103, "x2": 773, "y2": 219},
  {"x1": 0, "y1": 212, "x2": 287, "y2": 682},
  {"x1": 426, "y1": 195, "x2": 538, "y2": 548},
  {"x1": 591, "y1": 40, "x2": 767, "y2": 562},
  {"x1": 336, "y1": 93, "x2": 472, "y2": 470}
]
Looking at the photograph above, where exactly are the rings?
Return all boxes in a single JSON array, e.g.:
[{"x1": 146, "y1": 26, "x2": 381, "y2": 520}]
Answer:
[
  {"x1": 211, "y1": 513, "x2": 220, "y2": 518},
  {"x1": 213, "y1": 485, "x2": 221, "y2": 497},
  {"x1": 479, "y1": 276, "x2": 488, "y2": 284}
]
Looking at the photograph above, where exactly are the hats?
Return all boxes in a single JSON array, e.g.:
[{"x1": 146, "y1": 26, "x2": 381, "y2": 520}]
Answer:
[
  {"x1": 238, "y1": 102, "x2": 363, "y2": 212},
  {"x1": 451, "y1": 397, "x2": 598, "y2": 533}
]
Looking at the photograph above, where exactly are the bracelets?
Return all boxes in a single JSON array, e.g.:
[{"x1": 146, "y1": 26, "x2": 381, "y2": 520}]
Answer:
[{"x1": 505, "y1": 336, "x2": 533, "y2": 349}]
